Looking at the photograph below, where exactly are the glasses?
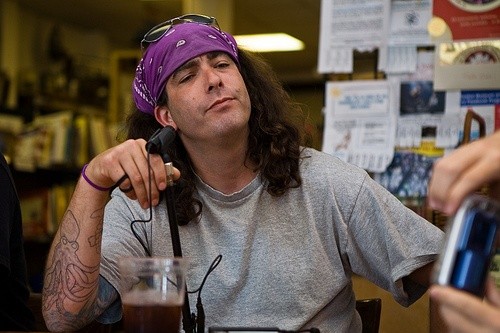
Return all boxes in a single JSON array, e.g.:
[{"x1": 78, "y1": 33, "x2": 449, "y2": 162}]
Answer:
[{"x1": 141, "y1": 14, "x2": 220, "y2": 59}]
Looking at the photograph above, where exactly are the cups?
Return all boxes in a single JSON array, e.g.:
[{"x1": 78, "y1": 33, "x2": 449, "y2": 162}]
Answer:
[{"x1": 118, "y1": 257, "x2": 184, "y2": 333}]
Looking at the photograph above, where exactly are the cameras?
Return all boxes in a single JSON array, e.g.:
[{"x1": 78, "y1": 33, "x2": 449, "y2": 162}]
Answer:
[{"x1": 431, "y1": 193, "x2": 500, "y2": 302}]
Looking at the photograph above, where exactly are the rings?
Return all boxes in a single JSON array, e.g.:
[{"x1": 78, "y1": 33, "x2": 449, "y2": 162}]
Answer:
[{"x1": 120, "y1": 185, "x2": 133, "y2": 193}]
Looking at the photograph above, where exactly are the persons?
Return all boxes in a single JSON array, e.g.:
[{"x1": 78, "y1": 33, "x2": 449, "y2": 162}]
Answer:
[
  {"x1": 0, "y1": 147, "x2": 38, "y2": 333},
  {"x1": 42, "y1": 14, "x2": 445, "y2": 333},
  {"x1": 429, "y1": 129, "x2": 500, "y2": 333}
]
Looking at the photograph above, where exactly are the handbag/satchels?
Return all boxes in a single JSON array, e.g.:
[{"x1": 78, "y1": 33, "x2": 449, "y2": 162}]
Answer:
[{"x1": 373, "y1": 152, "x2": 436, "y2": 197}]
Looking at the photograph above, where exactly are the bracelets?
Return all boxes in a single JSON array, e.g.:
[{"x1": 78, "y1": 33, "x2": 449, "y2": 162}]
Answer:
[{"x1": 82, "y1": 164, "x2": 113, "y2": 191}]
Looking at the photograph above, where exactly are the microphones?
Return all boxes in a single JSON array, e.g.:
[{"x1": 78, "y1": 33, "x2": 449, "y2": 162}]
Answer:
[{"x1": 146, "y1": 125, "x2": 176, "y2": 153}]
